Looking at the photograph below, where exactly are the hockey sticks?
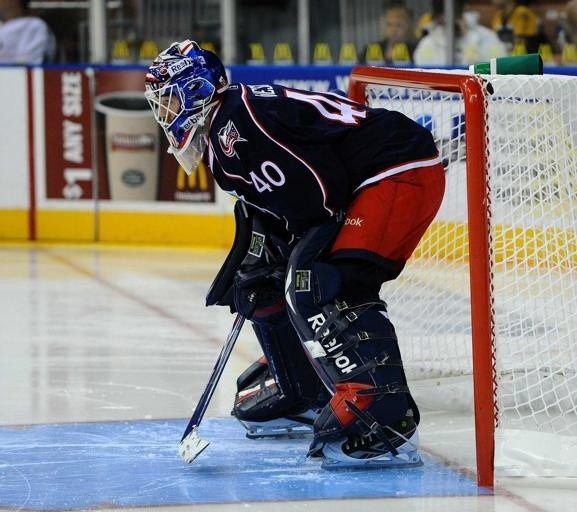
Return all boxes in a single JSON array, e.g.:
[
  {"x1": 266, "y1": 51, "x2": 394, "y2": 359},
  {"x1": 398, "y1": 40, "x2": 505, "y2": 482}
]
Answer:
[{"x1": 176, "y1": 313, "x2": 246, "y2": 464}]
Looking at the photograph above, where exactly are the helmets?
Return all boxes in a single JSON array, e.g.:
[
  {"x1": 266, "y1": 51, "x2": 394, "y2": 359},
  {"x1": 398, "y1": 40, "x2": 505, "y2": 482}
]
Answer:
[{"x1": 143, "y1": 38, "x2": 228, "y2": 176}]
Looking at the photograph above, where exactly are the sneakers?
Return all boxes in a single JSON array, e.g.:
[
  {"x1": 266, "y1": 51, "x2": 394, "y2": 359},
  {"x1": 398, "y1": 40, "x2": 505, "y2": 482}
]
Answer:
[{"x1": 323, "y1": 416, "x2": 417, "y2": 464}]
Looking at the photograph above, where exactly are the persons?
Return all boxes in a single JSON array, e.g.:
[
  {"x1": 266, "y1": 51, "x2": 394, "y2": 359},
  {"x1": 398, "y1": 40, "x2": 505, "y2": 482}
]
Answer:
[
  {"x1": 0, "y1": 0, "x2": 57, "y2": 65},
  {"x1": 142, "y1": 37, "x2": 444, "y2": 454},
  {"x1": 355, "y1": 0, "x2": 576, "y2": 68}
]
